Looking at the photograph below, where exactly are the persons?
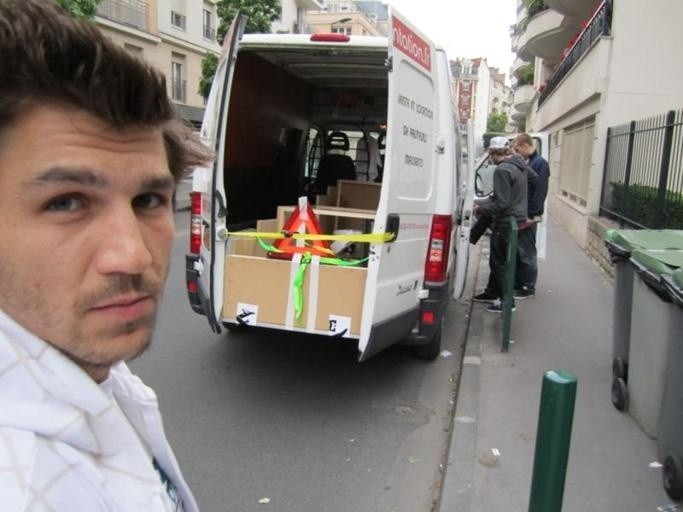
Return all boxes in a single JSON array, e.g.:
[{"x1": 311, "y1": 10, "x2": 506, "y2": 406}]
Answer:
[
  {"x1": 2, "y1": 1, "x2": 199, "y2": 512},
  {"x1": 472, "y1": 137, "x2": 540, "y2": 315},
  {"x1": 513, "y1": 133, "x2": 550, "y2": 301}
]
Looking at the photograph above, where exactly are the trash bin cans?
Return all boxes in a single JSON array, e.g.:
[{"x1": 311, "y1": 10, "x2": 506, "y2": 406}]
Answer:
[{"x1": 600, "y1": 228, "x2": 683, "y2": 501}]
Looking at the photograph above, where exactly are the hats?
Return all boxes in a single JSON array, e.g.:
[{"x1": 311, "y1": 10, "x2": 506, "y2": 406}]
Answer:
[{"x1": 489, "y1": 136, "x2": 510, "y2": 149}]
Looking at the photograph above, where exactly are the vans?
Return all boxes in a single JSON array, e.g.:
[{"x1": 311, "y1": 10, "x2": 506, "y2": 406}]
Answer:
[{"x1": 183, "y1": 5, "x2": 553, "y2": 369}]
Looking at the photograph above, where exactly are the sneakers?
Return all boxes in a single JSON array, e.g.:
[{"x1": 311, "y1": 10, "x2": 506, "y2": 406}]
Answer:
[
  {"x1": 487, "y1": 301, "x2": 516, "y2": 312},
  {"x1": 472, "y1": 294, "x2": 500, "y2": 303},
  {"x1": 513, "y1": 286, "x2": 534, "y2": 299}
]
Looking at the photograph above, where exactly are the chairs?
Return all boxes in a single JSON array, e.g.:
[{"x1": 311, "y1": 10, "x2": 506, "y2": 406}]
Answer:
[{"x1": 314, "y1": 132, "x2": 355, "y2": 194}]
